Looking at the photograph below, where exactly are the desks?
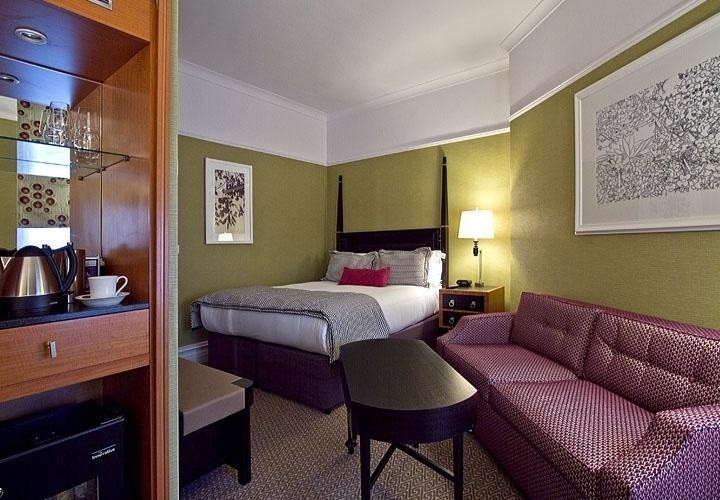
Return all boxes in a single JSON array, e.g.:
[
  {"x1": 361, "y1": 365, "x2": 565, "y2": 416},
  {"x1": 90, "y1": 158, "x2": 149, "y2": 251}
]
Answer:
[{"x1": 179, "y1": 359, "x2": 255, "y2": 493}]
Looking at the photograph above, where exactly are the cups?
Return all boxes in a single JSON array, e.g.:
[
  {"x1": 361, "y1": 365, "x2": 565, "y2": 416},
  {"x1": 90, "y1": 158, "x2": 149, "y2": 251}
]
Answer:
[
  {"x1": 88, "y1": 274, "x2": 129, "y2": 300},
  {"x1": 36, "y1": 100, "x2": 103, "y2": 168},
  {"x1": 49, "y1": 248, "x2": 87, "y2": 304}
]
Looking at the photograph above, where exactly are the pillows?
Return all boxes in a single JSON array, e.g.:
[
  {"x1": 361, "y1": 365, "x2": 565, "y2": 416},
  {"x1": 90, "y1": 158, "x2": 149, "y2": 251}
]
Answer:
[{"x1": 323, "y1": 246, "x2": 445, "y2": 289}]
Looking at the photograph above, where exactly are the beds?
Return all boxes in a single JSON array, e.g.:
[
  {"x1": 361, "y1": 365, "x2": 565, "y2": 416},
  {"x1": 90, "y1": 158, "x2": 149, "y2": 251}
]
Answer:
[{"x1": 188, "y1": 153, "x2": 454, "y2": 412}]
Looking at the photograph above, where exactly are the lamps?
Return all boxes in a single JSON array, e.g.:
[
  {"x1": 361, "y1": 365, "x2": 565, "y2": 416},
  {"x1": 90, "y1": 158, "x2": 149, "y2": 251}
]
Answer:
[{"x1": 458, "y1": 209, "x2": 497, "y2": 286}]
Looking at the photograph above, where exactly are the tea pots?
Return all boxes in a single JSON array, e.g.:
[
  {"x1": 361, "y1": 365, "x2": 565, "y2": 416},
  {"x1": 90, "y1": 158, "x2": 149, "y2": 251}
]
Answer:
[{"x1": 0, "y1": 244, "x2": 78, "y2": 318}]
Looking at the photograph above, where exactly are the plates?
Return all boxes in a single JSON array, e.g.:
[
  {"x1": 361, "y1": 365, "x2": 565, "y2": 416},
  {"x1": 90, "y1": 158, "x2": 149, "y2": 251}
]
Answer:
[{"x1": 75, "y1": 292, "x2": 131, "y2": 308}]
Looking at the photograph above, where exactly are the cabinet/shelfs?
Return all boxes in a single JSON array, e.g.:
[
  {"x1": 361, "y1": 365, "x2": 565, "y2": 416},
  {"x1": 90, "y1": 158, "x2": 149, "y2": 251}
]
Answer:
[
  {"x1": 438, "y1": 282, "x2": 506, "y2": 332},
  {"x1": 0, "y1": 0, "x2": 171, "y2": 500}
]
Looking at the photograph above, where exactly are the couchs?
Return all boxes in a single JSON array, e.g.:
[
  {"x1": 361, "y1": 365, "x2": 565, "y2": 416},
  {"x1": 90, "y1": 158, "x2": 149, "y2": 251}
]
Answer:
[{"x1": 437, "y1": 291, "x2": 720, "y2": 500}]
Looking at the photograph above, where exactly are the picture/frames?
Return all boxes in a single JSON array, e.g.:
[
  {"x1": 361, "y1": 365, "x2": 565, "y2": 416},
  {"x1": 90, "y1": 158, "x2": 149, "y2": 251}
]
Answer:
[
  {"x1": 203, "y1": 157, "x2": 256, "y2": 245},
  {"x1": 573, "y1": 12, "x2": 720, "y2": 235}
]
